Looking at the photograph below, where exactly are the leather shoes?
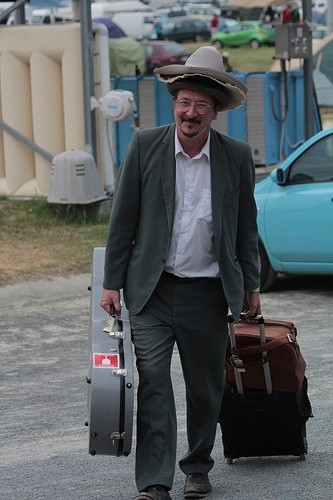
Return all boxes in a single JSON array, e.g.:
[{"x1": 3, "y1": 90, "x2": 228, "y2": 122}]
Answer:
[
  {"x1": 180, "y1": 473, "x2": 212, "y2": 500},
  {"x1": 134, "y1": 486, "x2": 172, "y2": 500}
]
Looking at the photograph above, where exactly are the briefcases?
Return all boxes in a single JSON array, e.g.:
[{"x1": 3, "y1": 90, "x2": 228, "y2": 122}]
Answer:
[{"x1": 85, "y1": 246, "x2": 135, "y2": 457}]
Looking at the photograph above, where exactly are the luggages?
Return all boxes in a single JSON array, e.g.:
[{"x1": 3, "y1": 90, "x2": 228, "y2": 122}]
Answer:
[{"x1": 217, "y1": 312, "x2": 315, "y2": 465}]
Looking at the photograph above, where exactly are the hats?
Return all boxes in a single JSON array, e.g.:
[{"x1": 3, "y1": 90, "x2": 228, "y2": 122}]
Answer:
[{"x1": 153, "y1": 45, "x2": 249, "y2": 112}]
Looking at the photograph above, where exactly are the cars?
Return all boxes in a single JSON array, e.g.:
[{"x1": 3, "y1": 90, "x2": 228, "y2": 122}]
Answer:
[
  {"x1": 0, "y1": 0, "x2": 329, "y2": 44},
  {"x1": 141, "y1": 40, "x2": 193, "y2": 76},
  {"x1": 254, "y1": 128, "x2": 333, "y2": 294},
  {"x1": 210, "y1": 20, "x2": 277, "y2": 50}
]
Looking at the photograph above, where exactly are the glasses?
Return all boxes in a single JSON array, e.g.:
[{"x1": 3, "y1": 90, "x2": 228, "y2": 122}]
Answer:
[{"x1": 173, "y1": 97, "x2": 215, "y2": 115}]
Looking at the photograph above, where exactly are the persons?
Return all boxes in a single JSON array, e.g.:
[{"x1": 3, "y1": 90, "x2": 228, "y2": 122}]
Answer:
[
  {"x1": 210, "y1": 14, "x2": 218, "y2": 37},
  {"x1": 261, "y1": 5, "x2": 300, "y2": 23},
  {"x1": 100, "y1": 46, "x2": 260, "y2": 500}
]
